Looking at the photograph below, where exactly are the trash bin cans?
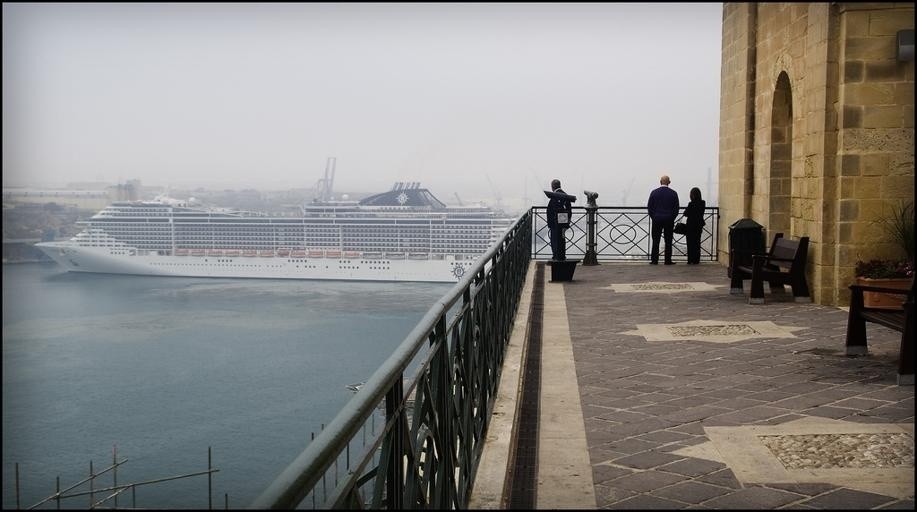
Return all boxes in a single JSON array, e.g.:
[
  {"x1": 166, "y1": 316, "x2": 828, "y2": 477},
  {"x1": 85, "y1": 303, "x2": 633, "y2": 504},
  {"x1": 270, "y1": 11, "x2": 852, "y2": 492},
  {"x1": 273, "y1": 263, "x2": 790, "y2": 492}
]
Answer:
[{"x1": 727, "y1": 218, "x2": 764, "y2": 278}]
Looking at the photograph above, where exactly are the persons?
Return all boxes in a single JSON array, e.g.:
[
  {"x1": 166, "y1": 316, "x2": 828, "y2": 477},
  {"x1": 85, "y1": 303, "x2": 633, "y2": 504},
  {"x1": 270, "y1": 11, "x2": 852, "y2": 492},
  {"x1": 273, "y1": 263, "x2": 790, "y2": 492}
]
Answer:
[
  {"x1": 545, "y1": 178, "x2": 572, "y2": 267},
  {"x1": 645, "y1": 174, "x2": 679, "y2": 266},
  {"x1": 682, "y1": 187, "x2": 706, "y2": 266}
]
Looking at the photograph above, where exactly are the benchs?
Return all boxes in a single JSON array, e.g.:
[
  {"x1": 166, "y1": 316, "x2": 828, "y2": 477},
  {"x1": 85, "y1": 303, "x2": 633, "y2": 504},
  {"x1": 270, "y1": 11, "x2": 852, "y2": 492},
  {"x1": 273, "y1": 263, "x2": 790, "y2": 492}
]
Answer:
[
  {"x1": 844, "y1": 285, "x2": 917, "y2": 385},
  {"x1": 730, "y1": 233, "x2": 812, "y2": 304}
]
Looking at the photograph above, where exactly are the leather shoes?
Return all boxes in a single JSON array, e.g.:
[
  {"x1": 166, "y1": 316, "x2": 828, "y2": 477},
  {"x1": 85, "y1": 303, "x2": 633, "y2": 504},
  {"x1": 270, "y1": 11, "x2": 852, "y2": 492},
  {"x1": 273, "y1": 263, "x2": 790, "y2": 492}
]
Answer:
[
  {"x1": 649, "y1": 261, "x2": 658, "y2": 264},
  {"x1": 664, "y1": 261, "x2": 676, "y2": 264}
]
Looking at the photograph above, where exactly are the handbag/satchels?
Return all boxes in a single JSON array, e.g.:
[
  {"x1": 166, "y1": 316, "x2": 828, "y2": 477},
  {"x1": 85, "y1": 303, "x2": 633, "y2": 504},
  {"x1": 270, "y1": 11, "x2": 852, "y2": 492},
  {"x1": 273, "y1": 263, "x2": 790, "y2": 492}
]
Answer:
[{"x1": 674, "y1": 222, "x2": 687, "y2": 234}]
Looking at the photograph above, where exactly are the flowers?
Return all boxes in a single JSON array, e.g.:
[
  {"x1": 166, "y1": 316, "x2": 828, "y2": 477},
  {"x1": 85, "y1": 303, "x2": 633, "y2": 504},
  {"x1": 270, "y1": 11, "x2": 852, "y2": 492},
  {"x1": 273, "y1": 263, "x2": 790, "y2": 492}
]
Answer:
[{"x1": 855, "y1": 259, "x2": 913, "y2": 280}]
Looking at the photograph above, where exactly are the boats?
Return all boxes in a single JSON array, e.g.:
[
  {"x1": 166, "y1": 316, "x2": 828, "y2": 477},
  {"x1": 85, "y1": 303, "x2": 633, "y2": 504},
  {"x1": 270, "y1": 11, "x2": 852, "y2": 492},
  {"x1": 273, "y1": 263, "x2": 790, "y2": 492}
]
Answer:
[{"x1": 342, "y1": 376, "x2": 425, "y2": 411}]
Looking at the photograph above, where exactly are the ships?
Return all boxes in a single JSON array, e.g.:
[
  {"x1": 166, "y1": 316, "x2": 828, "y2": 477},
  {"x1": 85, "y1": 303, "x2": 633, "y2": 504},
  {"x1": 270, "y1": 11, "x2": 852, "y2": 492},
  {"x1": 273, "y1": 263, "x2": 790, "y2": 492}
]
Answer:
[{"x1": 29, "y1": 154, "x2": 519, "y2": 286}]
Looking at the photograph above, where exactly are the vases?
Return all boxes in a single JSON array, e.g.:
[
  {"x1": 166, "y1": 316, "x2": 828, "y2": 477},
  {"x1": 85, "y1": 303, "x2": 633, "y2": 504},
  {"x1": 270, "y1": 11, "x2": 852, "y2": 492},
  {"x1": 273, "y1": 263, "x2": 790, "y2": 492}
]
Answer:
[{"x1": 855, "y1": 277, "x2": 913, "y2": 309}]
